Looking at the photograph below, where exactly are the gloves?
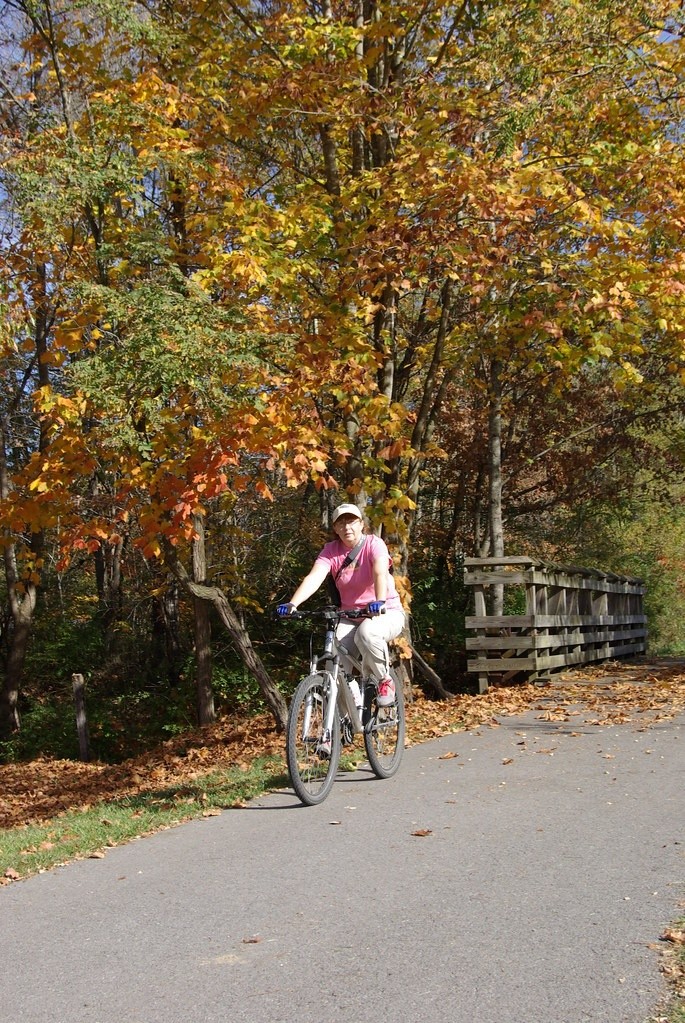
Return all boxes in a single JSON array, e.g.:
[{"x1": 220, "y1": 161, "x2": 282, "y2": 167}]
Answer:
[
  {"x1": 366, "y1": 600, "x2": 386, "y2": 616},
  {"x1": 276, "y1": 602, "x2": 297, "y2": 615}
]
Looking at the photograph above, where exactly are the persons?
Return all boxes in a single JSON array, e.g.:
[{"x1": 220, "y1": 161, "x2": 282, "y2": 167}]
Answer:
[{"x1": 277, "y1": 504, "x2": 405, "y2": 760}]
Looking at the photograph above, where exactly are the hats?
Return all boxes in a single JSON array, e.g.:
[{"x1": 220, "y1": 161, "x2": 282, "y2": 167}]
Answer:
[{"x1": 332, "y1": 504, "x2": 362, "y2": 523}]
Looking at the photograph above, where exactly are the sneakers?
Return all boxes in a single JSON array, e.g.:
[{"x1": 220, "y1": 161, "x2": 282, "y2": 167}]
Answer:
[
  {"x1": 376, "y1": 675, "x2": 395, "y2": 707},
  {"x1": 314, "y1": 742, "x2": 331, "y2": 760}
]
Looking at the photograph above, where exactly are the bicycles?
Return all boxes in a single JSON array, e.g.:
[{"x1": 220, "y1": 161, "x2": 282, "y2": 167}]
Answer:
[{"x1": 280, "y1": 608, "x2": 405, "y2": 805}]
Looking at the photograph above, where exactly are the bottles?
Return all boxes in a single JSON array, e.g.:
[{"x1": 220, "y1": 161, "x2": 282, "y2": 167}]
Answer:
[{"x1": 347, "y1": 674, "x2": 363, "y2": 707}]
